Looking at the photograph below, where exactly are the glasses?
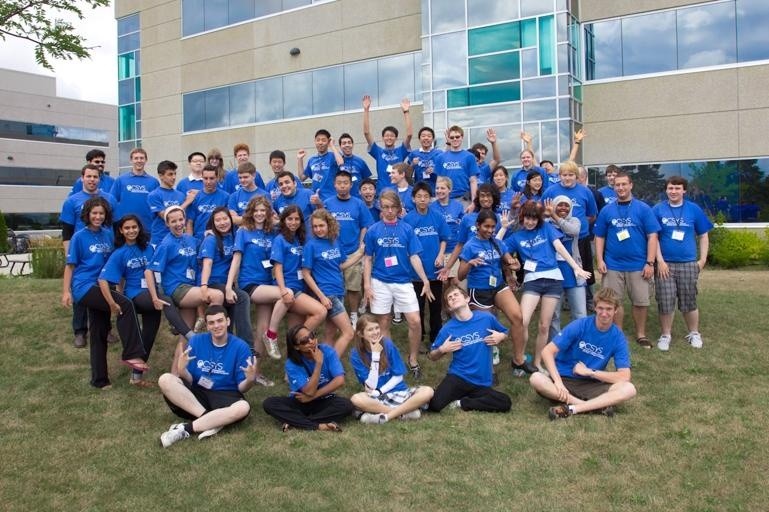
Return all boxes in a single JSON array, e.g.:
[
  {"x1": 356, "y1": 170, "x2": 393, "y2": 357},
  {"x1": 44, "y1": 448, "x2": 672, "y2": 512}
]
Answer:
[
  {"x1": 95, "y1": 161, "x2": 105, "y2": 163},
  {"x1": 450, "y1": 136, "x2": 460, "y2": 139},
  {"x1": 295, "y1": 331, "x2": 316, "y2": 345}
]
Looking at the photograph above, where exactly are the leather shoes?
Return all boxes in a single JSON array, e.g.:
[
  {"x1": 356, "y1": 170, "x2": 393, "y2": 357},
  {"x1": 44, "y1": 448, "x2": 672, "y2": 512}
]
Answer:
[
  {"x1": 75, "y1": 335, "x2": 86, "y2": 347},
  {"x1": 107, "y1": 333, "x2": 118, "y2": 343}
]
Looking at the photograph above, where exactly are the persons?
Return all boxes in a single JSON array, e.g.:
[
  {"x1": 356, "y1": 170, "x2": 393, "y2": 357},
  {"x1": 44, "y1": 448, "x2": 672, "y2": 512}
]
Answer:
[{"x1": 59, "y1": 98, "x2": 713, "y2": 451}]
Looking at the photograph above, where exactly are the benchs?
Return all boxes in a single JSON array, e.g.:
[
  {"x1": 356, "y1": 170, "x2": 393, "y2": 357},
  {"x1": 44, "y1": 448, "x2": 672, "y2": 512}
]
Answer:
[{"x1": 8, "y1": 259, "x2": 32, "y2": 276}]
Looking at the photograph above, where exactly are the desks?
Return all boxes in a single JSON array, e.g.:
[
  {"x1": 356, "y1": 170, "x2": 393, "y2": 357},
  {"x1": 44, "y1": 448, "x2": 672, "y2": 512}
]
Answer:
[{"x1": 0, "y1": 252, "x2": 10, "y2": 268}]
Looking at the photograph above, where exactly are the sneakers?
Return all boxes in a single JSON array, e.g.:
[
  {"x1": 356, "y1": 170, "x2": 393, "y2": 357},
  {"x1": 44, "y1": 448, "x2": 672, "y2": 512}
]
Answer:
[
  {"x1": 194, "y1": 318, "x2": 206, "y2": 332},
  {"x1": 256, "y1": 374, "x2": 274, "y2": 387},
  {"x1": 548, "y1": 407, "x2": 618, "y2": 418},
  {"x1": 351, "y1": 298, "x2": 368, "y2": 328},
  {"x1": 361, "y1": 412, "x2": 421, "y2": 423},
  {"x1": 198, "y1": 427, "x2": 223, "y2": 440},
  {"x1": 419, "y1": 342, "x2": 429, "y2": 354},
  {"x1": 161, "y1": 423, "x2": 190, "y2": 449},
  {"x1": 636, "y1": 335, "x2": 703, "y2": 350},
  {"x1": 129, "y1": 379, "x2": 154, "y2": 389},
  {"x1": 263, "y1": 329, "x2": 282, "y2": 359},
  {"x1": 407, "y1": 356, "x2": 420, "y2": 371},
  {"x1": 392, "y1": 313, "x2": 403, "y2": 325}
]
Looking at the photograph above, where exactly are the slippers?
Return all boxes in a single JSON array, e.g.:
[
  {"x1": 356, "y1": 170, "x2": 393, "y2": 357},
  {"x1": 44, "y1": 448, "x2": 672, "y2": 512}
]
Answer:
[
  {"x1": 326, "y1": 421, "x2": 342, "y2": 432},
  {"x1": 122, "y1": 358, "x2": 148, "y2": 372},
  {"x1": 282, "y1": 423, "x2": 290, "y2": 432}
]
